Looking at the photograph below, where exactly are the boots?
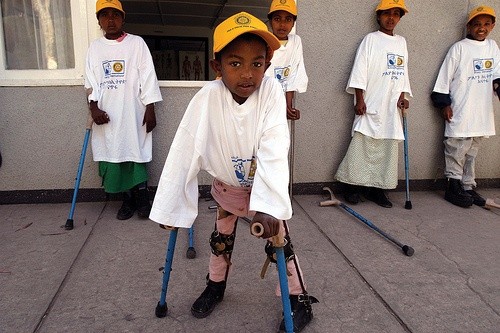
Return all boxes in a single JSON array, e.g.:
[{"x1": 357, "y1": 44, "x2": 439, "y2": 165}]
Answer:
[
  {"x1": 445, "y1": 178, "x2": 472, "y2": 207},
  {"x1": 466, "y1": 189, "x2": 486, "y2": 206}
]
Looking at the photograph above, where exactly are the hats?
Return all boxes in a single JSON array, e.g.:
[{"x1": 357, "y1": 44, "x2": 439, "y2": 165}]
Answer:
[
  {"x1": 213, "y1": 12, "x2": 281, "y2": 59},
  {"x1": 375, "y1": 0, "x2": 409, "y2": 16},
  {"x1": 465, "y1": 7, "x2": 496, "y2": 25},
  {"x1": 96, "y1": 0, "x2": 125, "y2": 20},
  {"x1": 268, "y1": 0, "x2": 297, "y2": 21}
]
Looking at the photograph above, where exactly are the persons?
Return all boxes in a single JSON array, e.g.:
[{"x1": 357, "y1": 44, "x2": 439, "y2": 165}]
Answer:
[
  {"x1": 84, "y1": 0, "x2": 163, "y2": 220},
  {"x1": 150, "y1": 12, "x2": 320, "y2": 333},
  {"x1": 431, "y1": 7, "x2": 500, "y2": 208},
  {"x1": 263, "y1": 0, "x2": 309, "y2": 121},
  {"x1": 333, "y1": 0, "x2": 415, "y2": 208}
]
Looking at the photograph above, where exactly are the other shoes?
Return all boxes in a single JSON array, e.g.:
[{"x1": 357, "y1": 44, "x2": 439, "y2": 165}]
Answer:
[
  {"x1": 191, "y1": 274, "x2": 227, "y2": 318},
  {"x1": 117, "y1": 197, "x2": 137, "y2": 220},
  {"x1": 344, "y1": 186, "x2": 360, "y2": 204},
  {"x1": 363, "y1": 187, "x2": 393, "y2": 208},
  {"x1": 281, "y1": 294, "x2": 317, "y2": 333},
  {"x1": 137, "y1": 188, "x2": 151, "y2": 216}
]
